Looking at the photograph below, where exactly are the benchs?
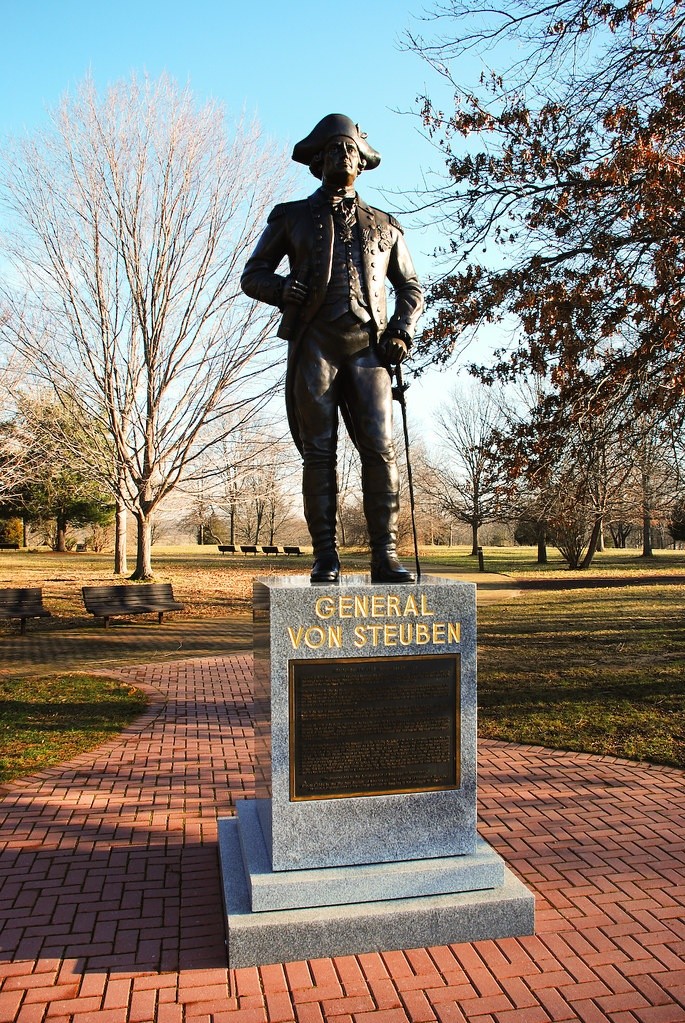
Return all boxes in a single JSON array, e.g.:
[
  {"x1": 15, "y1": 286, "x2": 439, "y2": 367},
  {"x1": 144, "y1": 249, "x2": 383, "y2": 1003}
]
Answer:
[
  {"x1": 262, "y1": 546, "x2": 283, "y2": 557},
  {"x1": 283, "y1": 547, "x2": 302, "y2": 556},
  {"x1": 82, "y1": 583, "x2": 185, "y2": 628},
  {"x1": 0, "y1": 543, "x2": 20, "y2": 551},
  {"x1": 0, "y1": 587, "x2": 51, "y2": 636},
  {"x1": 240, "y1": 545, "x2": 261, "y2": 556},
  {"x1": 218, "y1": 545, "x2": 239, "y2": 556}
]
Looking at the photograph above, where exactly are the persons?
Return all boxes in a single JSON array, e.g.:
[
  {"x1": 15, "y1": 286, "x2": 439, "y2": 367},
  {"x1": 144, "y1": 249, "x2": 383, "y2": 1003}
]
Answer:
[{"x1": 242, "y1": 115, "x2": 424, "y2": 583}]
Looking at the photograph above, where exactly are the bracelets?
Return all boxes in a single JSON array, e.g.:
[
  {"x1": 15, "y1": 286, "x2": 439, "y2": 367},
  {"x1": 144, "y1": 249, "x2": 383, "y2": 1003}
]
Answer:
[{"x1": 390, "y1": 329, "x2": 415, "y2": 350}]
